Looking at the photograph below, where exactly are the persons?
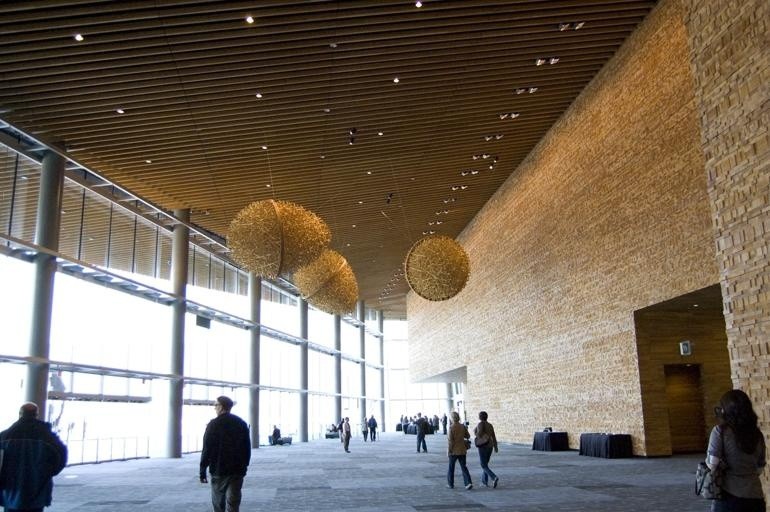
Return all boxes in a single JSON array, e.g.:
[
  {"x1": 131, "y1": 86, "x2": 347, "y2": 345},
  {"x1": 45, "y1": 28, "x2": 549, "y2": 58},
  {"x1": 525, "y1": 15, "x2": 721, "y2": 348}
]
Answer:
[
  {"x1": 446, "y1": 412, "x2": 473, "y2": 489},
  {"x1": 709, "y1": 389, "x2": 766, "y2": 512},
  {"x1": 199, "y1": 396, "x2": 251, "y2": 512},
  {"x1": 473, "y1": 411, "x2": 499, "y2": 488},
  {"x1": 328, "y1": 414, "x2": 377, "y2": 453},
  {"x1": 272, "y1": 425, "x2": 280, "y2": 446},
  {"x1": 399, "y1": 412, "x2": 447, "y2": 453},
  {"x1": 0, "y1": 402, "x2": 67, "y2": 512}
]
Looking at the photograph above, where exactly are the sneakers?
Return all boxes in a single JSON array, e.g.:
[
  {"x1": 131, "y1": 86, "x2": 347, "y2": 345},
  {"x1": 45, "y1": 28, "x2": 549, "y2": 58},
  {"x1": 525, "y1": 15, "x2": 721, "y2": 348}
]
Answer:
[
  {"x1": 479, "y1": 483, "x2": 487, "y2": 487},
  {"x1": 493, "y1": 476, "x2": 498, "y2": 488},
  {"x1": 465, "y1": 484, "x2": 472, "y2": 489}
]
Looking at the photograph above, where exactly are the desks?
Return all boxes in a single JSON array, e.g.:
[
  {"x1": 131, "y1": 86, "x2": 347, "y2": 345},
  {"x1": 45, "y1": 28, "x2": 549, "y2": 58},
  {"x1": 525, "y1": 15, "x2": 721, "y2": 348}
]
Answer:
[
  {"x1": 532, "y1": 432, "x2": 570, "y2": 451},
  {"x1": 268, "y1": 422, "x2": 440, "y2": 446},
  {"x1": 578, "y1": 431, "x2": 634, "y2": 460}
]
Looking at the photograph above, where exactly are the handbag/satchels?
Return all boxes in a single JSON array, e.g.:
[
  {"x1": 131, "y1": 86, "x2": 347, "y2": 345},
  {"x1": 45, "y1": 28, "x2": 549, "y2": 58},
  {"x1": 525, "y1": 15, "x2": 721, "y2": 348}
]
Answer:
[
  {"x1": 475, "y1": 434, "x2": 489, "y2": 447},
  {"x1": 696, "y1": 463, "x2": 723, "y2": 500},
  {"x1": 464, "y1": 440, "x2": 471, "y2": 449}
]
